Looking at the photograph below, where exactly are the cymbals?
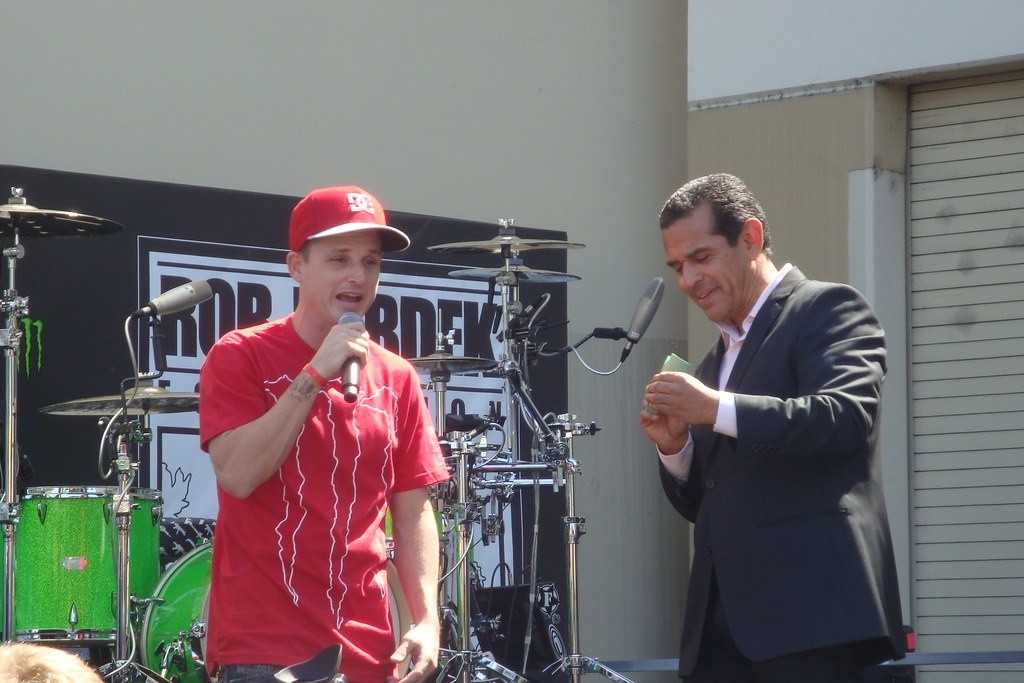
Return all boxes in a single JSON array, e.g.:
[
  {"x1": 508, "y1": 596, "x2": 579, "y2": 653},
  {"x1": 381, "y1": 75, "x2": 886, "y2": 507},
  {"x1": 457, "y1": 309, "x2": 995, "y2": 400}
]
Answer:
[
  {"x1": 2, "y1": 185, "x2": 129, "y2": 243},
  {"x1": 425, "y1": 217, "x2": 587, "y2": 256},
  {"x1": 404, "y1": 345, "x2": 498, "y2": 374},
  {"x1": 36, "y1": 377, "x2": 201, "y2": 416},
  {"x1": 447, "y1": 257, "x2": 583, "y2": 283}
]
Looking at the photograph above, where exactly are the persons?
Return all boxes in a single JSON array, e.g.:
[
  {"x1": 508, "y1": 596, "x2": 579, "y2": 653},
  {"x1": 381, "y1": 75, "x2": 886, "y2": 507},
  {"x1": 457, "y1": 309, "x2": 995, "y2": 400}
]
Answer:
[
  {"x1": 639, "y1": 174, "x2": 907, "y2": 683},
  {"x1": 1, "y1": 642, "x2": 106, "y2": 683},
  {"x1": 198, "y1": 186, "x2": 450, "y2": 683}
]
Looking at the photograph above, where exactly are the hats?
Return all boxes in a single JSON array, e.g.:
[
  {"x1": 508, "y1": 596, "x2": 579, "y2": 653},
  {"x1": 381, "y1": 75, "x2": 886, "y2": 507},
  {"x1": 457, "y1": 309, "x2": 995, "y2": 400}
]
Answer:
[{"x1": 288, "y1": 185, "x2": 411, "y2": 255}]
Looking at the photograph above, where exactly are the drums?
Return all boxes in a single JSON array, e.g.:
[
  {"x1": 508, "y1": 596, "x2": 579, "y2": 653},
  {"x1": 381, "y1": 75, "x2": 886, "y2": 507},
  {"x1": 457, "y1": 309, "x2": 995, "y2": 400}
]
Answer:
[
  {"x1": 0, "y1": 482, "x2": 166, "y2": 648},
  {"x1": 197, "y1": 556, "x2": 414, "y2": 681},
  {"x1": 383, "y1": 503, "x2": 472, "y2": 683},
  {"x1": 125, "y1": 536, "x2": 215, "y2": 683}
]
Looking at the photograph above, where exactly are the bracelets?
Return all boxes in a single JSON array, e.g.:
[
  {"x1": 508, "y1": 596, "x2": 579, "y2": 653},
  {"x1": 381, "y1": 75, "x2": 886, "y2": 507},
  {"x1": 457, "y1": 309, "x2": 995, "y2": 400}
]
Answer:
[{"x1": 303, "y1": 365, "x2": 326, "y2": 387}]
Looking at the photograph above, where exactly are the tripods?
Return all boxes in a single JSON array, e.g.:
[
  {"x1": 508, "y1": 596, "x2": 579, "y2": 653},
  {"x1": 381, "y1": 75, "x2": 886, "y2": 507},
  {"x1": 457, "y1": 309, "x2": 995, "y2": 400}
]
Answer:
[
  {"x1": 435, "y1": 424, "x2": 635, "y2": 683},
  {"x1": 97, "y1": 413, "x2": 173, "y2": 683}
]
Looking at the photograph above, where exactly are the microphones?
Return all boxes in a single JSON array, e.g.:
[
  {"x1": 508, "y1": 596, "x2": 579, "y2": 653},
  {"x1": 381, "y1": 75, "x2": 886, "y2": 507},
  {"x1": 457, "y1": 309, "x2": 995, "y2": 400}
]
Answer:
[
  {"x1": 338, "y1": 312, "x2": 365, "y2": 403},
  {"x1": 132, "y1": 279, "x2": 214, "y2": 319},
  {"x1": 498, "y1": 296, "x2": 541, "y2": 343},
  {"x1": 620, "y1": 277, "x2": 666, "y2": 364},
  {"x1": 464, "y1": 422, "x2": 490, "y2": 442}
]
